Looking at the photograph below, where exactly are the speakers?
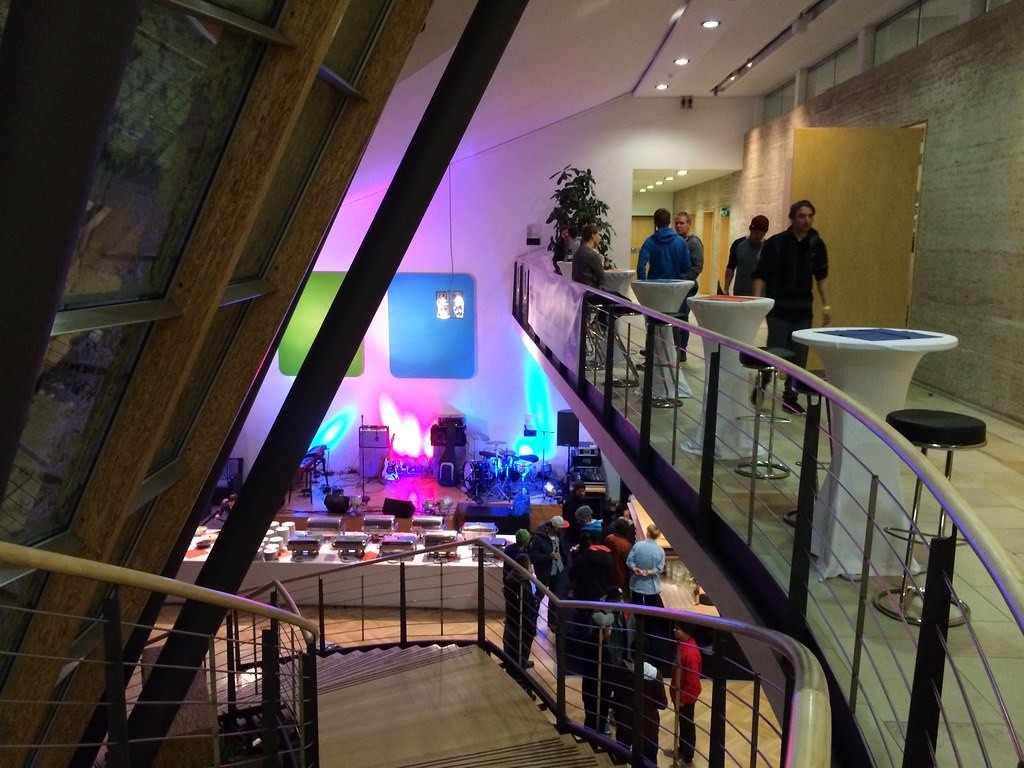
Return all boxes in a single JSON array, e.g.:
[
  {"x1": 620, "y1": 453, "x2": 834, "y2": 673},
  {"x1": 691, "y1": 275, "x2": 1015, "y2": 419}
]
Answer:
[
  {"x1": 358, "y1": 425, "x2": 390, "y2": 477},
  {"x1": 383, "y1": 497, "x2": 415, "y2": 517},
  {"x1": 324, "y1": 495, "x2": 350, "y2": 513},
  {"x1": 557, "y1": 409, "x2": 580, "y2": 447},
  {"x1": 430, "y1": 425, "x2": 467, "y2": 446}
]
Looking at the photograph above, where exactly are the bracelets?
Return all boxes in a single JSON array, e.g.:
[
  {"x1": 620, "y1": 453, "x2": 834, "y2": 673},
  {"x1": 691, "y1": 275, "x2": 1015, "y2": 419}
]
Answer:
[{"x1": 823, "y1": 306, "x2": 832, "y2": 309}]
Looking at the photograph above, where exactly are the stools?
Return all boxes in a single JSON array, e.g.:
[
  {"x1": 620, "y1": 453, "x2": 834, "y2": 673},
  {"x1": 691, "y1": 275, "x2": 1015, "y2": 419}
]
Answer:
[
  {"x1": 583, "y1": 289, "x2": 685, "y2": 408},
  {"x1": 871, "y1": 409, "x2": 988, "y2": 627},
  {"x1": 736, "y1": 346, "x2": 830, "y2": 527}
]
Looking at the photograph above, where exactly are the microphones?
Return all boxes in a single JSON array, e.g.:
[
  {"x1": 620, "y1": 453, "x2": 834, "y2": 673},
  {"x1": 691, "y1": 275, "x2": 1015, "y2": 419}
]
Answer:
[
  {"x1": 524, "y1": 425, "x2": 527, "y2": 431},
  {"x1": 375, "y1": 432, "x2": 378, "y2": 441}
]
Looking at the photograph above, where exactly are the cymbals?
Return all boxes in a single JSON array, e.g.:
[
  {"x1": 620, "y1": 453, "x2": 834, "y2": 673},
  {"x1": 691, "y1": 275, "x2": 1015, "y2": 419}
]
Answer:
[
  {"x1": 466, "y1": 429, "x2": 490, "y2": 442},
  {"x1": 485, "y1": 441, "x2": 506, "y2": 446}
]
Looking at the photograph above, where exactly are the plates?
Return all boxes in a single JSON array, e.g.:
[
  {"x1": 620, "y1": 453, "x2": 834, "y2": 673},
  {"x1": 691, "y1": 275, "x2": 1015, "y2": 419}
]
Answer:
[{"x1": 250, "y1": 521, "x2": 296, "y2": 563}]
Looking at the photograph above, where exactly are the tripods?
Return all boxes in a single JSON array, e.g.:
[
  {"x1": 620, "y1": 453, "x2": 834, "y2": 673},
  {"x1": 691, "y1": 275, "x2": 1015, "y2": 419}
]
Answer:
[{"x1": 486, "y1": 444, "x2": 539, "y2": 502}]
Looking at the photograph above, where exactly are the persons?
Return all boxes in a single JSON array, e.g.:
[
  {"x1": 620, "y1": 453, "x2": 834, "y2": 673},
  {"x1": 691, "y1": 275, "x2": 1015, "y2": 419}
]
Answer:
[
  {"x1": 722, "y1": 215, "x2": 769, "y2": 369},
  {"x1": 752, "y1": 200, "x2": 833, "y2": 415},
  {"x1": 636, "y1": 208, "x2": 689, "y2": 361},
  {"x1": 550, "y1": 224, "x2": 634, "y2": 329},
  {"x1": 663, "y1": 622, "x2": 702, "y2": 768},
  {"x1": 502, "y1": 480, "x2": 668, "y2": 768},
  {"x1": 674, "y1": 212, "x2": 703, "y2": 361}
]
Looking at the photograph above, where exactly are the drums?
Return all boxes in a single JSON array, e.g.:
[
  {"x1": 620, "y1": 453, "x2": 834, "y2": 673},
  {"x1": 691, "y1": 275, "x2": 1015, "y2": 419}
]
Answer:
[
  {"x1": 499, "y1": 458, "x2": 520, "y2": 484},
  {"x1": 465, "y1": 459, "x2": 496, "y2": 490}
]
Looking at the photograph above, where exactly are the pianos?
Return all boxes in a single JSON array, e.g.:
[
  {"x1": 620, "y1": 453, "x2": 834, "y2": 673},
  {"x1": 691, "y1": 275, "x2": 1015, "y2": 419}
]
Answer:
[{"x1": 286, "y1": 443, "x2": 329, "y2": 506}]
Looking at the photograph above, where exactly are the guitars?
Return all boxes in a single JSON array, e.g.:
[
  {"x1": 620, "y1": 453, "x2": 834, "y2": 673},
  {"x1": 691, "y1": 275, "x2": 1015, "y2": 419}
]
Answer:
[{"x1": 382, "y1": 432, "x2": 399, "y2": 481}]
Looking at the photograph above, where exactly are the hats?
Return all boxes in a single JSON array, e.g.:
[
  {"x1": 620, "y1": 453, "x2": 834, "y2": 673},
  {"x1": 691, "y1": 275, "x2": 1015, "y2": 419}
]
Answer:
[
  {"x1": 614, "y1": 516, "x2": 633, "y2": 531},
  {"x1": 592, "y1": 613, "x2": 614, "y2": 626},
  {"x1": 607, "y1": 586, "x2": 624, "y2": 597},
  {"x1": 550, "y1": 516, "x2": 570, "y2": 530},
  {"x1": 575, "y1": 505, "x2": 593, "y2": 522},
  {"x1": 516, "y1": 529, "x2": 532, "y2": 547}
]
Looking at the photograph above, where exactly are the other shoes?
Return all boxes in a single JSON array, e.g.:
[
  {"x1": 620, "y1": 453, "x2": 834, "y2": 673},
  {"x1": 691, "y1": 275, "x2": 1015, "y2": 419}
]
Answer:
[
  {"x1": 527, "y1": 661, "x2": 534, "y2": 667},
  {"x1": 639, "y1": 349, "x2": 646, "y2": 357},
  {"x1": 636, "y1": 361, "x2": 646, "y2": 371},
  {"x1": 676, "y1": 348, "x2": 687, "y2": 362},
  {"x1": 664, "y1": 749, "x2": 674, "y2": 758},
  {"x1": 782, "y1": 399, "x2": 806, "y2": 417},
  {"x1": 751, "y1": 383, "x2": 765, "y2": 405},
  {"x1": 670, "y1": 759, "x2": 693, "y2": 768}
]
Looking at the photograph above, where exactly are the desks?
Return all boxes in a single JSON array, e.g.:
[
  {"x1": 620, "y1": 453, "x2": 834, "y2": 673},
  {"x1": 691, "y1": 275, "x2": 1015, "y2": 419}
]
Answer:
[
  {"x1": 629, "y1": 279, "x2": 695, "y2": 400},
  {"x1": 597, "y1": 269, "x2": 638, "y2": 366},
  {"x1": 789, "y1": 326, "x2": 959, "y2": 580},
  {"x1": 681, "y1": 295, "x2": 775, "y2": 463},
  {"x1": 162, "y1": 527, "x2": 516, "y2": 611},
  {"x1": 287, "y1": 459, "x2": 330, "y2": 504}
]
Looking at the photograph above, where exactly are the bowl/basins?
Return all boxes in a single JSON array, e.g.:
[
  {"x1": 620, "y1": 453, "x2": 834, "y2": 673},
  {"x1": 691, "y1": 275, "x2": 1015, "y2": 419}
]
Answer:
[{"x1": 195, "y1": 526, "x2": 207, "y2": 536}]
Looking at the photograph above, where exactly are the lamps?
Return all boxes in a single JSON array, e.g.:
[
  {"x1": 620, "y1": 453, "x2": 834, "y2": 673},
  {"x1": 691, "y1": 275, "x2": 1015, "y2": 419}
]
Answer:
[{"x1": 435, "y1": 157, "x2": 465, "y2": 321}]
